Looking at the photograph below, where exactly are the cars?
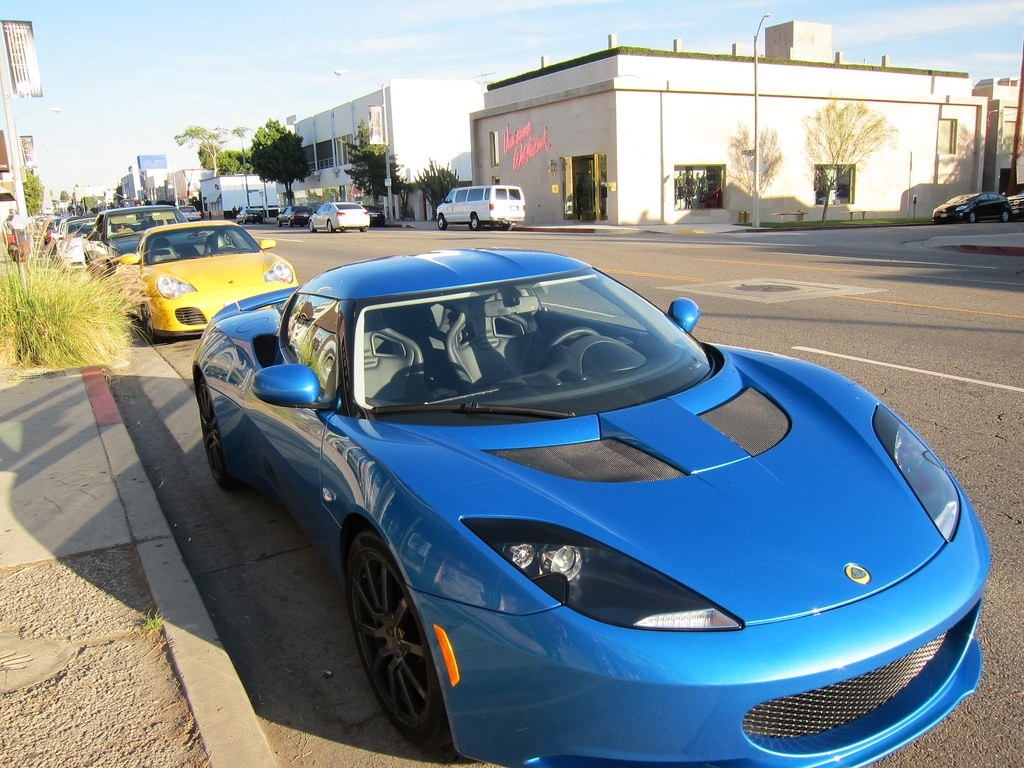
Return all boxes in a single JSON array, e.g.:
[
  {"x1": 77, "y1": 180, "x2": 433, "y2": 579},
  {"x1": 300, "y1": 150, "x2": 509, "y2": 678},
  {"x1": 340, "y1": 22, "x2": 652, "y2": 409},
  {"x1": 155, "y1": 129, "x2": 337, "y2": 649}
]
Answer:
[
  {"x1": 361, "y1": 205, "x2": 385, "y2": 228},
  {"x1": 82, "y1": 205, "x2": 190, "y2": 277},
  {"x1": 36, "y1": 214, "x2": 97, "y2": 268},
  {"x1": 931, "y1": 192, "x2": 1013, "y2": 223},
  {"x1": 308, "y1": 202, "x2": 370, "y2": 233},
  {"x1": 1007, "y1": 190, "x2": 1024, "y2": 219},
  {"x1": 276, "y1": 206, "x2": 314, "y2": 227},
  {"x1": 237, "y1": 209, "x2": 263, "y2": 224}
]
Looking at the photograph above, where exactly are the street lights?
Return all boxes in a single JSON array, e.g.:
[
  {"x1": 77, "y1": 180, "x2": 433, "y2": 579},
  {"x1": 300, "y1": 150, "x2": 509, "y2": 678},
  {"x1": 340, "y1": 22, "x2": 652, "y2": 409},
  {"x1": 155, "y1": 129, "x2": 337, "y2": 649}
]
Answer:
[
  {"x1": 14, "y1": 107, "x2": 60, "y2": 163},
  {"x1": 334, "y1": 70, "x2": 394, "y2": 221},
  {"x1": 152, "y1": 160, "x2": 179, "y2": 209},
  {"x1": 754, "y1": 12, "x2": 771, "y2": 228}
]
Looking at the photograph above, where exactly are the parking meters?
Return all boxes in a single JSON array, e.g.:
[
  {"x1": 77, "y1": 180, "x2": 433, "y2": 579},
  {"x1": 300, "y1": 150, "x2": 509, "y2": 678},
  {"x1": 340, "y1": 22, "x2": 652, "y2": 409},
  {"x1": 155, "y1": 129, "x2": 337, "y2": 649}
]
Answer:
[
  {"x1": 3, "y1": 209, "x2": 31, "y2": 294},
  {"x1": 913, "y1": 195, "x2": 917, "y2": 219}
]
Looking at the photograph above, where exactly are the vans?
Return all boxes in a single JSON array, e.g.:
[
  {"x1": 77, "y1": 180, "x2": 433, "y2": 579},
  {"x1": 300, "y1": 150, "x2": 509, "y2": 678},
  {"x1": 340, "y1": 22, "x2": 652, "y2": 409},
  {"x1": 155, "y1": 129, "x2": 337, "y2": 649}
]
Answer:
[{"x1": 436, "y1": 185, "x2": 526, "y2": 231}]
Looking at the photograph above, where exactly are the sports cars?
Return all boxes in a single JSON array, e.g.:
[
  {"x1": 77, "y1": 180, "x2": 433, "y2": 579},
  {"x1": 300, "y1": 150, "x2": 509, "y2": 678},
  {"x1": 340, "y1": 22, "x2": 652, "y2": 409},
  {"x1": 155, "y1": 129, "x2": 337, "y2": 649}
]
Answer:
[
  {"x1": 116, "y1": 221, "x2": 299, "y2": 341},
  {"x1": 193, "y1": 248, "x2": 992, "y2": 768}
]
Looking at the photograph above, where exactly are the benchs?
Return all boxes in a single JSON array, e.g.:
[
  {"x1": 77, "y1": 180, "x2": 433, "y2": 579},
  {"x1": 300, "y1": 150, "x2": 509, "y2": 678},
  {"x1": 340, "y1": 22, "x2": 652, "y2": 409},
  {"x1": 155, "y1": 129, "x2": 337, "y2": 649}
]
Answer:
[
  {"x1": 842, "y1": 210, "x2": 874, "y2": 221},
  {"x1": 778, "y1": 209, "x2": 808, "y2": 224}
]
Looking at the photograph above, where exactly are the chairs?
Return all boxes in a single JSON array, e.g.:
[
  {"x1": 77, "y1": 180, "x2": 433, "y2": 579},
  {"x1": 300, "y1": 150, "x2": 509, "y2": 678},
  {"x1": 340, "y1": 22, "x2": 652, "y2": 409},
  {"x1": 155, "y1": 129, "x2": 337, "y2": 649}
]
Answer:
[
  {"x1": 363, "y1": 288, "x2": 548, "y2": 401},
  {"x1": 147, "y1": 231, "x2": 238, "y2": 264}
]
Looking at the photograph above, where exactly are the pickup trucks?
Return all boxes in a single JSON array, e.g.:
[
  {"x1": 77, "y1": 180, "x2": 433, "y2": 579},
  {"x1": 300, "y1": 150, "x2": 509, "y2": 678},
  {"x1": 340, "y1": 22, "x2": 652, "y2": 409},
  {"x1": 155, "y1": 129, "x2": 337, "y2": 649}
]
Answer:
[{"x1": 178, "y1": 206, "x2": 201, "y2": 220}]
